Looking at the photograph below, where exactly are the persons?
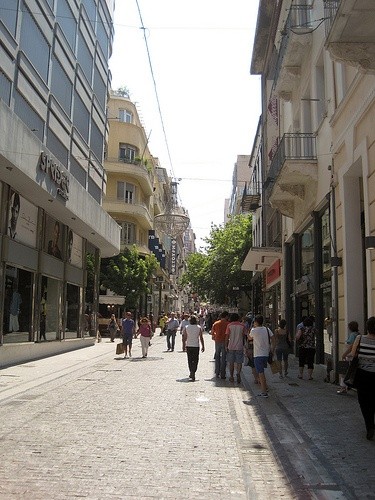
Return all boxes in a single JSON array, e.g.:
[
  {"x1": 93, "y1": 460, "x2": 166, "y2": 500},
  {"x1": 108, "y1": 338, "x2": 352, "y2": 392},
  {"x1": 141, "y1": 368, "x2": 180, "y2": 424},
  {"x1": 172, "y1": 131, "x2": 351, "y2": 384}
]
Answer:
[
  {"x1": 6, "y1": 192, "x2": 21, "y2": 241},
  {"x1": 84, "y1": 311, "x2": 95, "y2": 336},
  {"x1": 94, "y1": 307, "x2": 375, "y2": 443},
  {"x1": 67, "y1": 231, "x2": 74, "y2": 263},
  {"x1": 38, "y1": 294, "x2": 49, "y2": 341},
  {"x1": 48, "y1": 221, "x2": 63, "y2": 259}
]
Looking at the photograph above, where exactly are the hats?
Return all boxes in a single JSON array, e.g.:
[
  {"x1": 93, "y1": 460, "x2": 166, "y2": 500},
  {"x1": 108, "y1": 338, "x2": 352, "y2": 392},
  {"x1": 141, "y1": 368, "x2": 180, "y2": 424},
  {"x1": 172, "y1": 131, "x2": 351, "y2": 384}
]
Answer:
[{"x1": 171, "y1": 312, "x2": 176, "y2": 315}]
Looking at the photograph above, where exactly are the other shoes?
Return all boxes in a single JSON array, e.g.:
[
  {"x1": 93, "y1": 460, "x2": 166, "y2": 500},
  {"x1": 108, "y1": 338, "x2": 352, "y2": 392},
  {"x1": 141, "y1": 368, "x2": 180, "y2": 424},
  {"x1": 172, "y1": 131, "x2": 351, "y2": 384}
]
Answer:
[
  {"x1": 236, "y1": 374, "x2": 241, "y2": 383},
  {"x1": 229, "y1": 377, "x2": 234, "y2": 382},
  {"x1": 189, "y1": 371, "x2": 195, "y2": 381},
  {"x1": 337, "y1": 385, "x2": 348, "y2": 394},
  {"x1": 257, "y1": 392, "x2": 269, "y2": 398},
  {"x1": 221, "y1": 377, "x2": 226, "y2": 379},
  {"x1": 266, "y1": 386, "x2": 269, "y2": 391}
]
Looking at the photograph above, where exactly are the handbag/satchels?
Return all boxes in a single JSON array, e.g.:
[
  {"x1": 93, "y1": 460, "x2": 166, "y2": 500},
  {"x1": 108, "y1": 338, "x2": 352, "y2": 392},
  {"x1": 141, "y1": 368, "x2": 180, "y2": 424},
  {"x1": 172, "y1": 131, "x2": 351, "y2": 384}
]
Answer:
[
  {"x1": 268, "y1": 351, "x2": 273, "y2": 365},
  {"x1": 343, "y1": 335, "x2": 362, "y2": 387},
  {"x1": 116, "y1": 343, "x2": 125, "y2": 354},
  {"x1": 298, "y1": 335, "x2": 305, "y2": 345},
  {"x1": 271, "y1": 360, "x2": 281, "y2": 374}
]
